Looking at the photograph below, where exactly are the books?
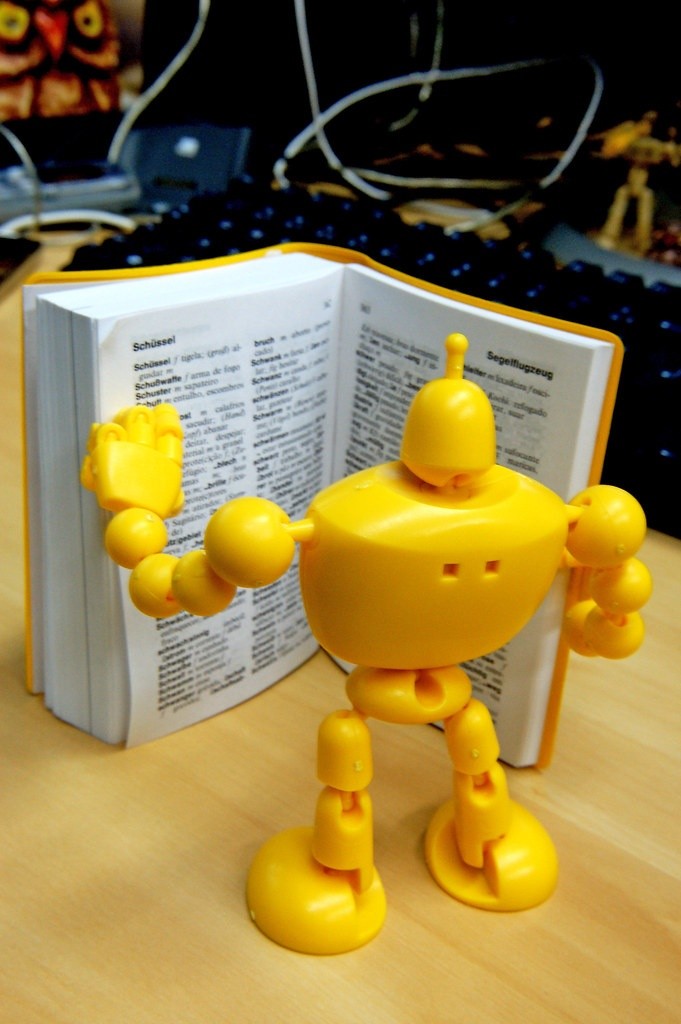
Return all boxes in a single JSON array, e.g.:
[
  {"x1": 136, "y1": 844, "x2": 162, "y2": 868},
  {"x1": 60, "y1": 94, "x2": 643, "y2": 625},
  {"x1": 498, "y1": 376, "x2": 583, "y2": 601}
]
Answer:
[{"x1": 19, "y1": 241, "x2": 624, "y2": 773}]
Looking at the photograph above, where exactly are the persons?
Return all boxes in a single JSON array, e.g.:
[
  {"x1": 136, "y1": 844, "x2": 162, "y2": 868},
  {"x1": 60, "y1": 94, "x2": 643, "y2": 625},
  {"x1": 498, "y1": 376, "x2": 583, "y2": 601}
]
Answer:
[{"x1": 79, "y1": 332, "x2": 653, "y2": 954}]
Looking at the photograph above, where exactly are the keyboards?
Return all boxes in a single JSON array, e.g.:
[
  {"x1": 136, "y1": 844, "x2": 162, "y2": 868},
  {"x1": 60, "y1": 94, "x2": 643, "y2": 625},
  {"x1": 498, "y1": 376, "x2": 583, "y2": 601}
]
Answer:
[{"x1": 62, "y1": 178, "x2": 681, "y2": 542}]
